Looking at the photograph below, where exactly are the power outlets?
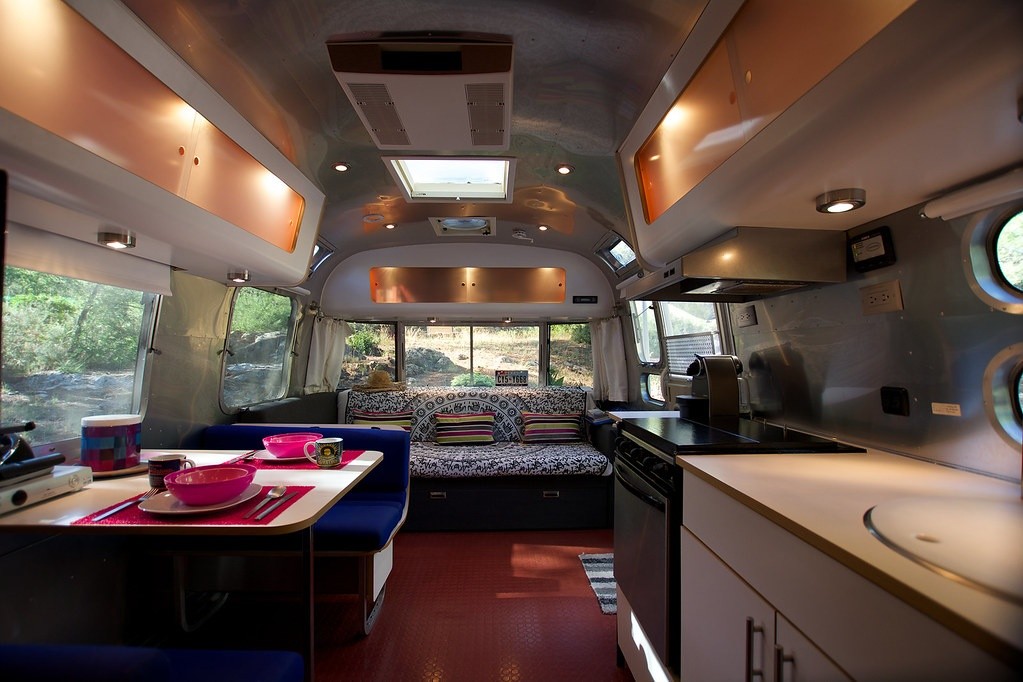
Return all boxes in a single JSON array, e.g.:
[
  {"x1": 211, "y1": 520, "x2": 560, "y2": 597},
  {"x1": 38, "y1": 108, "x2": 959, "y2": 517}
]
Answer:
[
  {"x1": 859, "y1": 280, "x2": 903, "y2": 313},
  {"x1": 736, "y1": 305, "x2": 758, "y2": 328}
]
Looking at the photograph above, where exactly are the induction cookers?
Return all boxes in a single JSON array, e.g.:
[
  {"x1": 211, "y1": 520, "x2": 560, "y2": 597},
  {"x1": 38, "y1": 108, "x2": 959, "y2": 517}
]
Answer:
[{"x1": 615, "y1": 417, "x2": 867, "y2": 496}]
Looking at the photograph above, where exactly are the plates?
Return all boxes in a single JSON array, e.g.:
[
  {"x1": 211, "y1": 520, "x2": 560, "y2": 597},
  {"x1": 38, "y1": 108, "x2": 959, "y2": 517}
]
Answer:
[
  {"x1": 73, "y1": 461, "x2": 149, "y2": 477},
  {"x1": 138, "y1": 483, "x2": 263, "y2": 514},
  {"x1": 252, "y1": 450, "x2": 316, "y2": 464}
]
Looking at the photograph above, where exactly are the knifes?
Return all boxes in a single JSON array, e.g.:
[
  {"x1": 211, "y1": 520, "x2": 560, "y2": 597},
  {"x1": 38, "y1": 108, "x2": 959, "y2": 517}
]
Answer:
[
  {"x1": 254, "y1": 490, "x2": 298, "y2": 520},
  {"x1": 229, "y1": 452, "x2": 255, "y2": 464}
]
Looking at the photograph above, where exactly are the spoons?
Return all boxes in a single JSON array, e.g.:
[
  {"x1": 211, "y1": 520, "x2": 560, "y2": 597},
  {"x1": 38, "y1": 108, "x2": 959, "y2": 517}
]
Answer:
[
  {"x1": 242, "y1": 485, "x2": 287, "y2": 519},
  {"x1": 243, "y1": 453, "x2": 256, "y2": 462}
]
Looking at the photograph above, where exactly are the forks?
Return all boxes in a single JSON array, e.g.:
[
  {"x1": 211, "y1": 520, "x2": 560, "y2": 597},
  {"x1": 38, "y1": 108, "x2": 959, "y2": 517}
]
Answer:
[{"x1": 91, "y1": 488, "x2": 159, "y2": 522}]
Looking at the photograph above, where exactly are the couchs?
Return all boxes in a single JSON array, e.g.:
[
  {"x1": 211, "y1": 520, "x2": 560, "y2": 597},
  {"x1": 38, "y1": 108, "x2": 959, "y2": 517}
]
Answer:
[{"x1": 337, "y1": 385, "x2": 615, "y2": 530}]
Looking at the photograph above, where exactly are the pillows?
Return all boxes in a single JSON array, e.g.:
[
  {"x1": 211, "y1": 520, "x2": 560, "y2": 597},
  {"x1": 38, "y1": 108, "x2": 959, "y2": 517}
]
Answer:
[
  {"x1": 433, "y1": 411, "x2": 499, "y2": 446},
  {"x1": 350, "y1": 409, "x2": 413, "y2": 432},
  {"x1": 521, "y1": 410, "x2": 588, "y2": 445}
]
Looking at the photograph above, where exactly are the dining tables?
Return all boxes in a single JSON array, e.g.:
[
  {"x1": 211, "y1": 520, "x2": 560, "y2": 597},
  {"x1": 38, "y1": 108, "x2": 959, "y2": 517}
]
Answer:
[{"x1": 0, "y1": 449, "x2": 383, "y2": 681}]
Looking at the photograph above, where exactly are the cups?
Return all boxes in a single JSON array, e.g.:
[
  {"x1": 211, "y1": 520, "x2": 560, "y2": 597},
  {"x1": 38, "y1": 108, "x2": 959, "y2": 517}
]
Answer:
[
  {"x1": 148, "y1": 454, "x2": 197, "y2": 493},
  {"x1": 303, "y1": 438, "x2": 343, "y2": 468},
  {"x1": 81, "y1": 413, "x2": 142, "y2": 471}
]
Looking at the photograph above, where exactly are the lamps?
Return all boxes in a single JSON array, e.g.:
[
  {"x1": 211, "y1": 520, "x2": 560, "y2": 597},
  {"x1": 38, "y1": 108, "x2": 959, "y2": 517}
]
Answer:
[
  {"x1": 98, "y1": 232, "x2": 136, "y2": 250},
  {"x1": 151, "y1": 347, "x2": 161, "y2": 355},
  {"x1": 428, "y1": 317, "x2": 437, "y2": 322},
  {"x1": 502, "y1": 318, "x2": 511, "y2": 322},
  {"x1": 292, "y1": 351, "x2": 298, "y2": 356},
  {"x1": 816, "y1": 188, "x2": 866, "y2": 214},
  {"x1": 226, "y1": 349, "x2": 234, "y2": 356},
  {"x1": 227, "y1": 272, "x2": 251, "y2": 282}
]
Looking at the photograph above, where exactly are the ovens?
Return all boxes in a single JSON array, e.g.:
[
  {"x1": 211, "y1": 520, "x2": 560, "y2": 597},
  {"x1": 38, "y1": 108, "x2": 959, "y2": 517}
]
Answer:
[{"x1": 613, "y1": 450, "x2": 680, "y2": 682}]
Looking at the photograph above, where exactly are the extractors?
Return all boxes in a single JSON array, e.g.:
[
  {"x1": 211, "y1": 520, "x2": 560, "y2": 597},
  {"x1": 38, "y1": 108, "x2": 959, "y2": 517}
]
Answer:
[{"x1": 626, "y1": 225, "x2": 848, "y2": 303}]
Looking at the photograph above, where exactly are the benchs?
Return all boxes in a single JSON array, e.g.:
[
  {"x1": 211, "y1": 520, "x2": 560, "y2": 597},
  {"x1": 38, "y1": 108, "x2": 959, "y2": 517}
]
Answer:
[
  {"x1": 0, "y1": 640, "x2": 306, "y2": 682},
  {"x1": 186, "y1": 421, "x2": 411, "y2": 635}
]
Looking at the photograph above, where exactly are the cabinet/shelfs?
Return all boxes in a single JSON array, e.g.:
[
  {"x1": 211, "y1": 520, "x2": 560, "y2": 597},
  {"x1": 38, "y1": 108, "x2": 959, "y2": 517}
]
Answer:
[{"x1": 675, "y1": 470, "x2": 1022, "y2": 681}]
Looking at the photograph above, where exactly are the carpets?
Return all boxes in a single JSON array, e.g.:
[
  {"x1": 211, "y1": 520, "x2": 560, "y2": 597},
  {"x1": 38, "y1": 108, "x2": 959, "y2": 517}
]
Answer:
[{"x1": 580, "y1": 552, "x2": 617, "y2": 615}]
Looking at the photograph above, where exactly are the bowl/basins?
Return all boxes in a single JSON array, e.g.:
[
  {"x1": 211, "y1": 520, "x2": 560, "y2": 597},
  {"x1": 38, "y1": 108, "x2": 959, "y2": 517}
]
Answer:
[
  {"x1": 261, "y1": 433, "x2": 323, "y2": 458},
  {"x1": 163, "y1": 463, "x2": 257, "y2": 507}
]
